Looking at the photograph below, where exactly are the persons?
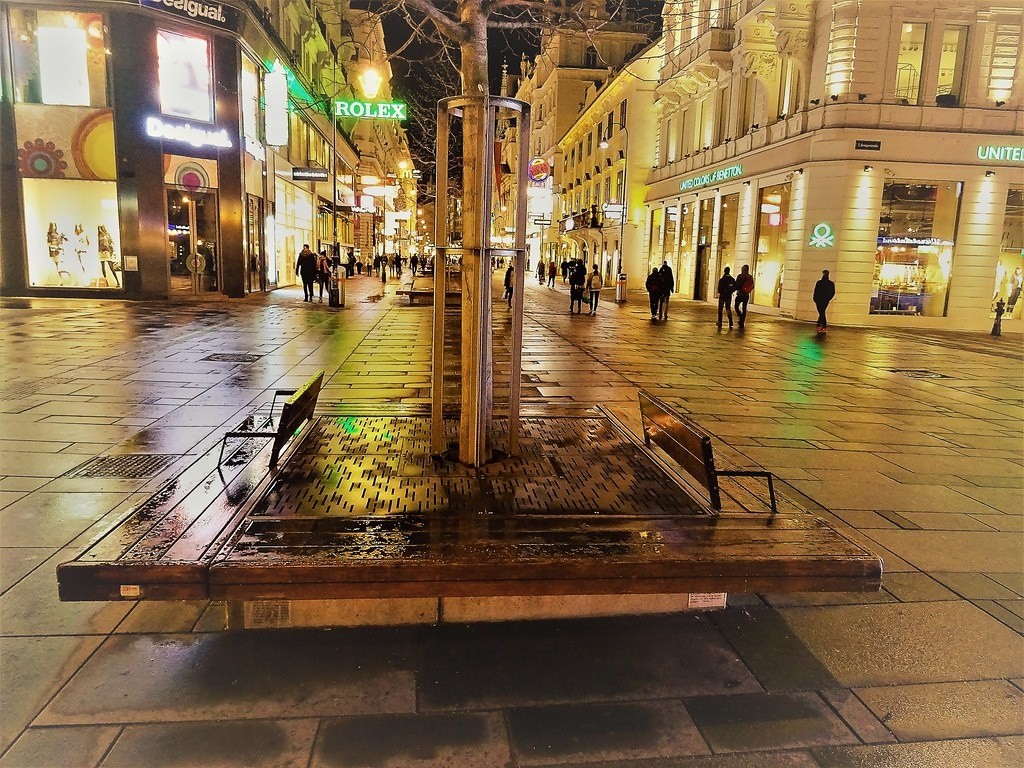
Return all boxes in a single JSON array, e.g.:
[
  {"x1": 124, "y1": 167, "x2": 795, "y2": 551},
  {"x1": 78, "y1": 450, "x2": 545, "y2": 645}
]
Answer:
[
  {"x1": 296, "y1": 244, "x2": 331, "y2": 303},
  {"x1": 735, "y1": 265, "x2": 753, "y2": 328},
  {"x1": 536, "y1": 256, "x2": 545, "y2": 282},
  {"x1": 491, "y1": 256, "x2": 515, "y2": 308},
  {"x1": 716, "y1": 267, "x2": 736, "y2": 328},
  {"x1": 992, "y1": 260, "x2": 1024, "y2": 312},
  {"x1": 561, "y1": 257, "x2": 586, "y2": 314},
  {"x1": 586, "y1": 264, "x2": 602, "y2": 314},
  {"x1": 546, "y1": 262, "x2": 555, "y2": 287},
  {"x1": 646, "y1": 261, "x2": 675, "y2": 319},
  {"x1": 813, "y1": 270, "x2": 836, "y2": 333},
  {"x1": 347, "y1": 252, "x2": 435, "y2": 283},
  {"x1": 47, "y1": 221, "x2": 120, "y2": 286}
]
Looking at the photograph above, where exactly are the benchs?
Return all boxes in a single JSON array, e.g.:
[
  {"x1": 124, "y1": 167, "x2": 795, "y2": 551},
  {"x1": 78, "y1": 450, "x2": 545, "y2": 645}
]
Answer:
[
  {"x1": 637, "y1": 386, "x2": 813, "y2": 515},
  {"x1": 215, "y1": 372, "x2": 327, "y2": 473}
]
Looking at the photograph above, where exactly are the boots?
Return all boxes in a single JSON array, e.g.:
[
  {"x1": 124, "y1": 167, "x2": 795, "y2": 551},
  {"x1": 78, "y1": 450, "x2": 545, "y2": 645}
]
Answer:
[{"x1": 319, "y1": 297, "x2": 322, "y2": 303}]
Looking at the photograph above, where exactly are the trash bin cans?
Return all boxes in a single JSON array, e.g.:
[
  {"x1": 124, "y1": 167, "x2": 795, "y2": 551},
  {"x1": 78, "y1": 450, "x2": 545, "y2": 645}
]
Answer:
[
  {"x1": 329, "y1": 265, "x2": 345, "y2": 306},
  {"x1": 615, "y1": 274, "x2": 627, "y2": 303}
]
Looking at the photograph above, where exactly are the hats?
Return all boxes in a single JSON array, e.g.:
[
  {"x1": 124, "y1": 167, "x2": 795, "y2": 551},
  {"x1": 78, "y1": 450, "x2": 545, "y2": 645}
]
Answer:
[{"x1": 320, "y1": 250, "x2": 326, "y2": 257}]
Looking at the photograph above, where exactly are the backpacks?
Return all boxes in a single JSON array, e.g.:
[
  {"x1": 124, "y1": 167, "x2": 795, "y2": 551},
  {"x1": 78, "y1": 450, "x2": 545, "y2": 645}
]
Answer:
[
  {"x1": 742, "y1": 274, "x2": 753, "y2": 293},
  {"x1": 591, "y1": 273, "x2": 601, "y2": 289}
]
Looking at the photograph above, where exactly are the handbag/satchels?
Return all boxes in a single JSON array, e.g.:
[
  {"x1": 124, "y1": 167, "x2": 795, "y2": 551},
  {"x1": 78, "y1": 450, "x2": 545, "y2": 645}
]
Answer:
[
  {"x1": 542, "y1": 276, "x2": 545, "y2": 282},
  {"x1": 535, "y1": 274, "x2": 537, "y2": 278},
  {"x1": 112, "y1": 261, "x2": 122, "y2": 271}
]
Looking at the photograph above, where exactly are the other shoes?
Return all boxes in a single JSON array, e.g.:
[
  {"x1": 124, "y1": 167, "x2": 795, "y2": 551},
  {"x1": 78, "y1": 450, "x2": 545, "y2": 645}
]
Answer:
[
  {"x1": 508, "y1": 306, "x2": 512, "y2": 309},
  {"x1": 303, "y1": 300, "x2": 308, "y2": 302},
  {"x1": 547, "y1": 285, "x2": 549, "y2": 286},
  {"x1": 588, "y1": 310, "x2": 592, "y2": 315},
  {"x1": 569, "y1": 311, "x2": 573, "y2": 313},
  {"x1": 818, "y1": 328, "x2": 827, "y2": 332},
  {"x1": 503, "y1": 297, "x2": 507, "y2": 299},
  {"x1": 552, "y1": 286, "x2": 554, "y2": 287},
  {"x1": 310, "y1": 298, "x2": 312, "y2": 301},
  {"x1": 593, "y1": 312, "x2": 597, "y2": 315},
  {"x1": 816, "y1": 326, "x2": 821, "y2": 330}
]
